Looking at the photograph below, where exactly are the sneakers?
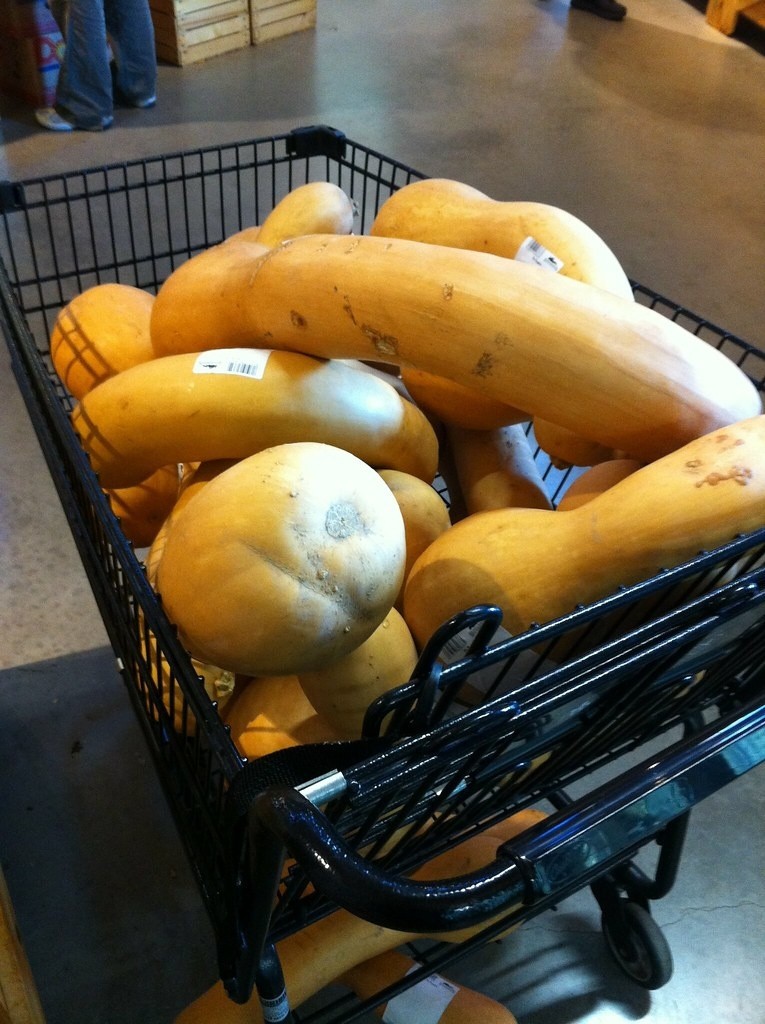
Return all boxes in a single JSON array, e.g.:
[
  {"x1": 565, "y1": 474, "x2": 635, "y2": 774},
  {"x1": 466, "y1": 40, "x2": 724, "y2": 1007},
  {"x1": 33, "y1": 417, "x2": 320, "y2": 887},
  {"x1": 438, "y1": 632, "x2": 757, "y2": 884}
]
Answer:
[
  {"x1": 35, "y1": 105, "x2": 115, "y2": 131},
  {"x1": 112, "y1": 80, "x2": 157, "y2": 110},
  {"x1": 570, "y1": 0, "x2": 627, "y2": 21}
]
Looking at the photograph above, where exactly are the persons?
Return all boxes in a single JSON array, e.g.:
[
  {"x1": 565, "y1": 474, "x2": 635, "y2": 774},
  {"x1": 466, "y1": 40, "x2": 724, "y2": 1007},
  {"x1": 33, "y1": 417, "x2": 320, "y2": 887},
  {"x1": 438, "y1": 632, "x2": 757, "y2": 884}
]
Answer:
[
  {"x1": 568, "y1": 0, "x2": 631, "y2": 22},
  {"x1": 34, "y1": 1, "x2": 159, "y2": 134}
]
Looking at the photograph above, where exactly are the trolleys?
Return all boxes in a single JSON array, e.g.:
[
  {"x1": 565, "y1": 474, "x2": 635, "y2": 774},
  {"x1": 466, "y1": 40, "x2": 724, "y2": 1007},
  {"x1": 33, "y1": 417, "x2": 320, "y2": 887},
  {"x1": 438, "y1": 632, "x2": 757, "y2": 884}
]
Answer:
[{"x1": 1, "y1": 124, "x2": 765, "y2": 1024}]
